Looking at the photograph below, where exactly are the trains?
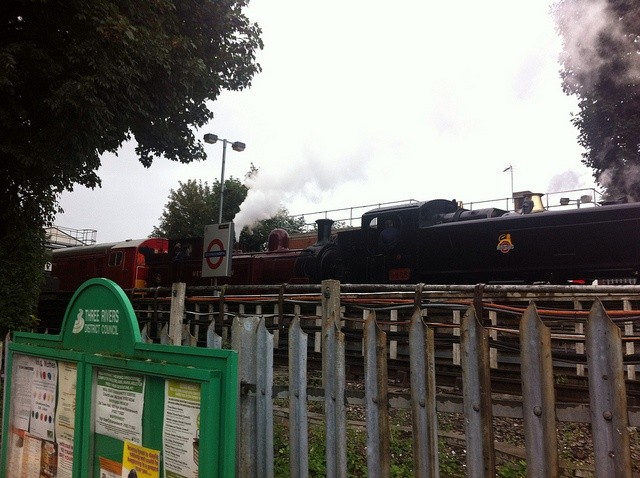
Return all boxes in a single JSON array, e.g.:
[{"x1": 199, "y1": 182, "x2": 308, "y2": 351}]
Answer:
[{"x1": 38, "y1": 200, "x2": 640, "y2": 403}]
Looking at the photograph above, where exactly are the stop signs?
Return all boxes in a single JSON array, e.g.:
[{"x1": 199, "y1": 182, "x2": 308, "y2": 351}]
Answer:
[{"x1": 202, "y1": 222, "x2": 231, "y2": 277}]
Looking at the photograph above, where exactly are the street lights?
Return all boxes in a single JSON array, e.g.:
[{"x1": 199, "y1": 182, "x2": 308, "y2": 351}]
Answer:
[
  {"x1": 203, "y1": 132, "x2": 245, "y2": 223},
  {"x1": 503, "y1": 165, "x2": 514, "y2": 197}
]
